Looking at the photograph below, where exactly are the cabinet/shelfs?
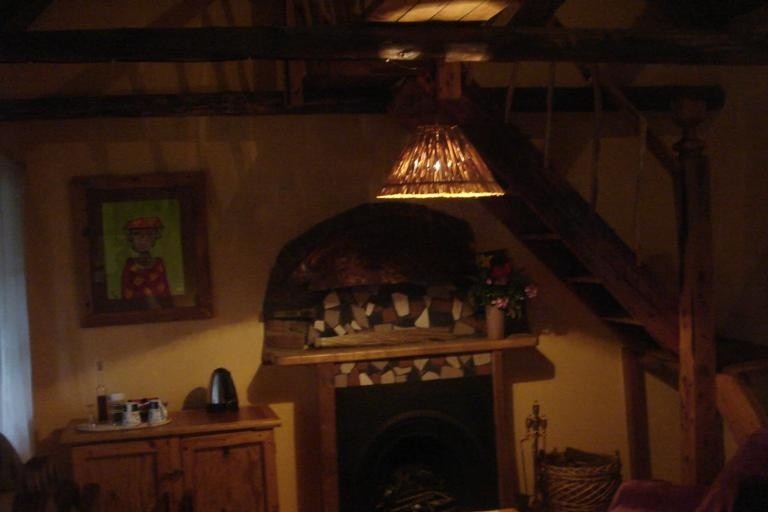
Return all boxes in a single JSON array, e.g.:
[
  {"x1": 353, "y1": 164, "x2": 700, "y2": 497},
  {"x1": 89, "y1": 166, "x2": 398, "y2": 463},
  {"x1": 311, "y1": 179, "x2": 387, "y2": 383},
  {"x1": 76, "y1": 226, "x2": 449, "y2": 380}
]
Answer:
[{"x1": 59, "y1": 404, "x2": 283, "y2": 512}]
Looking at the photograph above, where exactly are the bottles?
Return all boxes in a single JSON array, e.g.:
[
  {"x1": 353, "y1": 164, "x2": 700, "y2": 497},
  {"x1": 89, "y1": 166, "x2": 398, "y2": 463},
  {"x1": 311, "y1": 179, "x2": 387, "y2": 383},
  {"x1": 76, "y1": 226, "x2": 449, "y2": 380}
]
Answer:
[{"x1": 95, "y1": 360, "x2": 110, "y2": 424}]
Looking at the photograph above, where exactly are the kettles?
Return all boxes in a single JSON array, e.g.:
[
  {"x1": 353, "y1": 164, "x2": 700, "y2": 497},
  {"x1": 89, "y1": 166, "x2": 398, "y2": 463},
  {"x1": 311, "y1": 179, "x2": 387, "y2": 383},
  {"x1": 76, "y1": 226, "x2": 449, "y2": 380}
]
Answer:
[{"x1": 206, "y1": 368, "x2": 239, "y2": 413}]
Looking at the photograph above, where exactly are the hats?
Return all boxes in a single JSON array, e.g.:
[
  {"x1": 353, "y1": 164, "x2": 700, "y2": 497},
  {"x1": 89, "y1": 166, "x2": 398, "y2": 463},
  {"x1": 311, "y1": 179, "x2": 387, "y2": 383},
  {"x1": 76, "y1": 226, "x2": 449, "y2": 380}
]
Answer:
[
  {"x1": 472, "y1": 236, "x2": 505, "y2": 253},
  {"x1": 122, "y1": 216, "x2": 165, "y2": 230}
]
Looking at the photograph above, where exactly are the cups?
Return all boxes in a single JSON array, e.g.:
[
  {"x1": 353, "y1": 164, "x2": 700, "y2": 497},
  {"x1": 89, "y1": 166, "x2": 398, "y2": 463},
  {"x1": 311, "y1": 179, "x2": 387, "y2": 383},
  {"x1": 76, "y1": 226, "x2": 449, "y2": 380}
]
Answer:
[
  {"x1": 121, "y1": 402, "x2": 141, "y2": 428},
  {"x1": 148, "y1": 400, "x2": 168, "y2": 424}
]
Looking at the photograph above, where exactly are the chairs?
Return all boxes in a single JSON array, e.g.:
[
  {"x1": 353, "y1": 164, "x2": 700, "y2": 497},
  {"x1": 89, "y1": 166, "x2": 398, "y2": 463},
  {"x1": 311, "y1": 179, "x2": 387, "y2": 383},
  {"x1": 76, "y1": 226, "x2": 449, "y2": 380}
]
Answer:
[{"x1": 608, "y1": 428, "x2": 768, "y2": 512}]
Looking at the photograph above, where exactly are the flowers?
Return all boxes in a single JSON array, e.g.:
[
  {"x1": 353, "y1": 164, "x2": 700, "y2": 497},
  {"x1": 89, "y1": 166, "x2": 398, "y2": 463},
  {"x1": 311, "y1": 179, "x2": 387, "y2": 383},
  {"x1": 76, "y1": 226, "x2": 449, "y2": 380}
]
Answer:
[{"x1": 466, "y1": 253, "x2": 538, "y2": 320}]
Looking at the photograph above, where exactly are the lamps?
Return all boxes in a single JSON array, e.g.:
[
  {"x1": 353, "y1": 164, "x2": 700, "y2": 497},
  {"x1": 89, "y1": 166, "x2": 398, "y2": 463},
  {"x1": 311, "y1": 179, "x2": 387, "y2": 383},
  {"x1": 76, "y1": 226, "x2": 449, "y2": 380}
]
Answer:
[{"x1": 375, "y1": 42, "x2": 506, "y2": 200}]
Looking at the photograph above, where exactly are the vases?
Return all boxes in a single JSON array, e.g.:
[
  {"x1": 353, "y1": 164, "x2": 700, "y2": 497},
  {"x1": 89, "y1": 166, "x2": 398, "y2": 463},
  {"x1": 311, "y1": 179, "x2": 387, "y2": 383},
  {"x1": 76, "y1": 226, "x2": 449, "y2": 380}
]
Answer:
[{"x1": 485, "y1": 302, "x2": 506, "y2": 339}]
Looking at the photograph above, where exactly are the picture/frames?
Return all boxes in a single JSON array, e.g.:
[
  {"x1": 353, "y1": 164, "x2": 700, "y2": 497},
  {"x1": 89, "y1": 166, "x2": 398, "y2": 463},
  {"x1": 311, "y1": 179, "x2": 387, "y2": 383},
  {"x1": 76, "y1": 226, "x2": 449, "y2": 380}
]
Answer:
[{"x1": 65, "y1": 170, "x2": 213, "y2": 329}]
